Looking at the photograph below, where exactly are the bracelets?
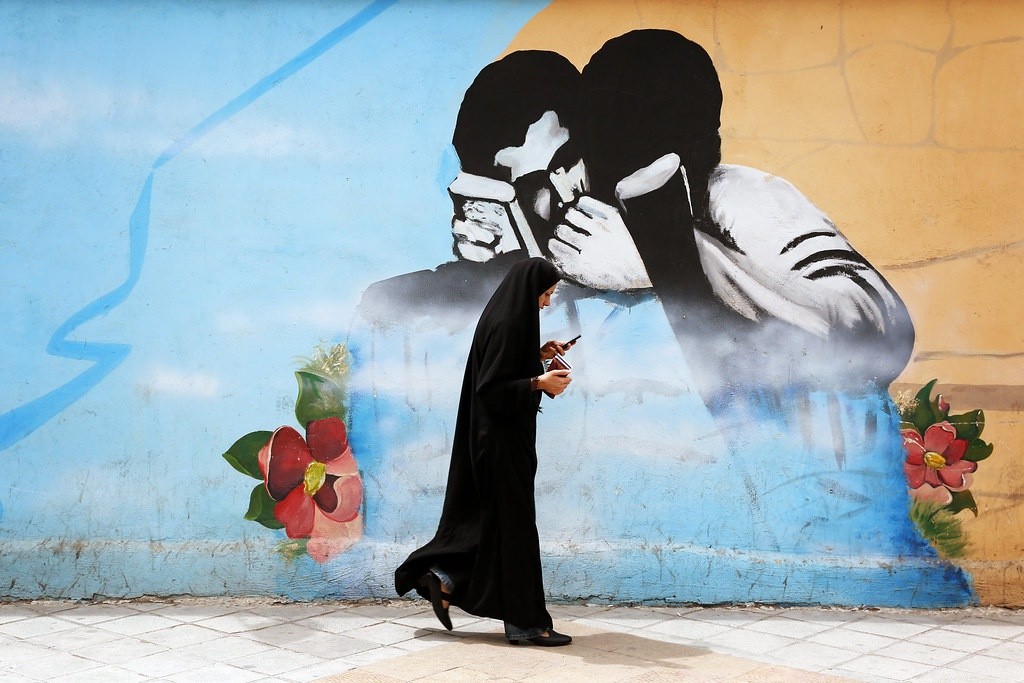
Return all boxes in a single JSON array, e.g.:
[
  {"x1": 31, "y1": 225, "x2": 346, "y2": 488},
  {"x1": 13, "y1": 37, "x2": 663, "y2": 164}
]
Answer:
[{"x1": 532, "y1": 376, "x2": 540, "y2": 391}]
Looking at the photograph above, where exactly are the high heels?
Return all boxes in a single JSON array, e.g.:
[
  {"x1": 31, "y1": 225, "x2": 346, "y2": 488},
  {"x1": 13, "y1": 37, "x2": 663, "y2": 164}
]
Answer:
[
  {"x1": 420, "y1": 572, "x2": 454, "y2": 631},
  {"x1": 508, "y1": 629, "x2": 571, "y2": 647}
]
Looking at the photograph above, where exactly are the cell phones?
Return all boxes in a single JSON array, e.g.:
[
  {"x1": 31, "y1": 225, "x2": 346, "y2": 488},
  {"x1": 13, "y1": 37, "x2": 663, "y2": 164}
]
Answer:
[{"x1": 556, "y1": 334, "x2": 582, "y2": 353}]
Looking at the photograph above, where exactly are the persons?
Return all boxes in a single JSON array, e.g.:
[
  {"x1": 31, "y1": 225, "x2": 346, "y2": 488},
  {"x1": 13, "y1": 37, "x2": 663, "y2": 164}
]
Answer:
[{"x1": 395, "y1": 257, "x2": 575, "y2": 647}]
